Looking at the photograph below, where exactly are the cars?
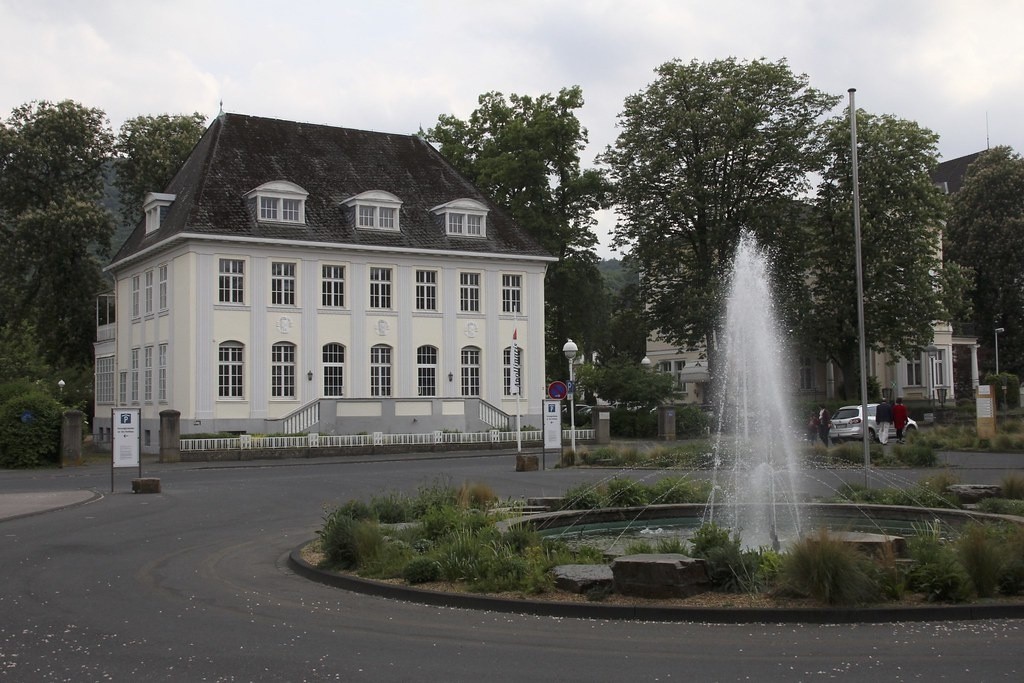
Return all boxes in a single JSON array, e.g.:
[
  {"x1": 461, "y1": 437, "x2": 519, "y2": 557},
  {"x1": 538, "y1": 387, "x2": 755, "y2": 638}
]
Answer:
[
  {"x1": 829, "y1": 403, "x2": 919, "y2": 447},
  {"x1": 560, "y1": 404, "x2": 657, "y2": 425}
]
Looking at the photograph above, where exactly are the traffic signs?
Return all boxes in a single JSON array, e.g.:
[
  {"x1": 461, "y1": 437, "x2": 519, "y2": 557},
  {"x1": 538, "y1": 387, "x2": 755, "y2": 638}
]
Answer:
[{"x1": 567, "y1": 380, "x2": 574, "y2": 400}]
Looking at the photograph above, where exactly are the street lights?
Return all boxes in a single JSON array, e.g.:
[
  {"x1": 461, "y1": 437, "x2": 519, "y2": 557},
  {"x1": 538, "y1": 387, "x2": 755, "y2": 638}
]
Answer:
[
  {"x1": 994, "y1": 327, "x2": 1006, "y2": 375},
  {"x1": 562, "y1": 338, "x2": 579, "y2": 455},
  {"x1": 58, "y1": 379, "x2": 65, "y2": 395}
]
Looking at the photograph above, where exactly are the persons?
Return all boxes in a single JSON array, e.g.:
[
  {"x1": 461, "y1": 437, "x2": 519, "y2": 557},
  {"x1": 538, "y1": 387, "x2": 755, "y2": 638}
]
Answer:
[
  {"x1": 808, "y1": 403, "x2": 832, "y2": 449},
  {"x1": 876, "y1": 398, "x2": 894, "y2": 445},
  {"x1": 892, "y1": 398, "x2": 909, "y2": 445}
]
